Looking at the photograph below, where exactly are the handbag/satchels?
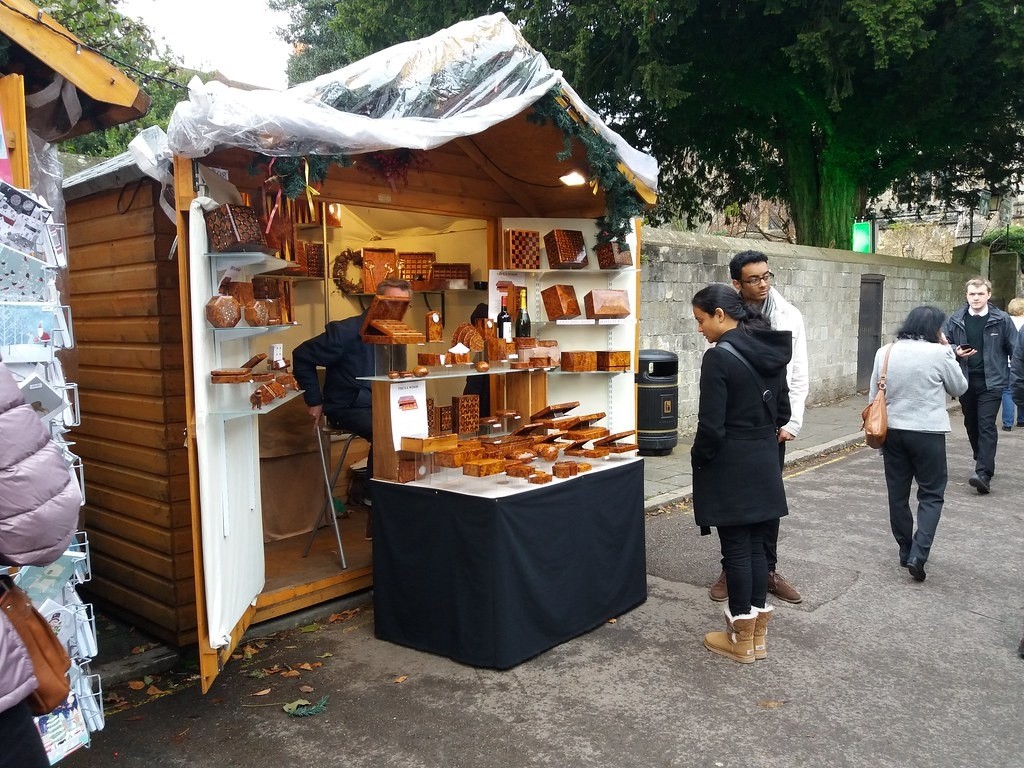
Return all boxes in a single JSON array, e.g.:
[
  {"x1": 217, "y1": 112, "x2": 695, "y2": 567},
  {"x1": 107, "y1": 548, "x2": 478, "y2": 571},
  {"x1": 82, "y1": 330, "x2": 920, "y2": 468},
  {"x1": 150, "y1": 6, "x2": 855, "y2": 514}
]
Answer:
[
  {"x1": 861, "y1": 343, "x2": 894, "y2": 449},
  {"x1": 0, "y1": 577, "x2": 71, "y2": 715}
]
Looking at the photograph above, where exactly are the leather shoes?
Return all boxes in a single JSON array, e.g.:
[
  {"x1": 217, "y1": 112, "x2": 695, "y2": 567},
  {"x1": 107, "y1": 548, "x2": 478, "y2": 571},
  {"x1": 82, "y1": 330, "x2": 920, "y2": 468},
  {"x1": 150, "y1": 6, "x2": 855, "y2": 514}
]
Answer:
[{"x1": 900, "y1": 560, "x2": 907, "y2": 567}]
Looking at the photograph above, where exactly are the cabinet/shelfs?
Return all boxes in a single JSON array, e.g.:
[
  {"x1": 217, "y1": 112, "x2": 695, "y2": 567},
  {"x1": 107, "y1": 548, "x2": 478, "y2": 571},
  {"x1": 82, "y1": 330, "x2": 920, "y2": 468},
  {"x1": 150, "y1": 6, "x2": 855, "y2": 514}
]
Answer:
[
  {"x1": 204, "y1": 252, "x2": 307, "y2": 540},
  {"x1": 498, "y1": 216, "x2": 640, "y2": 459},
  {"x1": 346, "y1": 291, "x2": 446, "y2": 331}
]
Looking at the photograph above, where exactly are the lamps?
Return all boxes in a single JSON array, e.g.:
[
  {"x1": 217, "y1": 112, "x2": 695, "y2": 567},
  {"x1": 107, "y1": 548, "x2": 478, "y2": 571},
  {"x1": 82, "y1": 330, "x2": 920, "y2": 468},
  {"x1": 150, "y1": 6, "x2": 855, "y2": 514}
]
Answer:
[{"x1": 558, "y1": 147, "x2": 587, "y2": 187}]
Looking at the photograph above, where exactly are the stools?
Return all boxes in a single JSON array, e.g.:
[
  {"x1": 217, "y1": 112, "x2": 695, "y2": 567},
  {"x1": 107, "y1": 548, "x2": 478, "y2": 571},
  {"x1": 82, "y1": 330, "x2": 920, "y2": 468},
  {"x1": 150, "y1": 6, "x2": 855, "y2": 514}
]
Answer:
[{"x1": 301, "y1": 365, "x2": 362, "y2": 569}]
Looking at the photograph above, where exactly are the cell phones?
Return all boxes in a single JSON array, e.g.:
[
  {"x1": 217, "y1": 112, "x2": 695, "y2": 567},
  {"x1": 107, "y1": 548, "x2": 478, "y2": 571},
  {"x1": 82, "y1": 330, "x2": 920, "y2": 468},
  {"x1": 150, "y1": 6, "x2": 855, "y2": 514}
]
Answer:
[{"x1": 961, "y1": 344, "x2": 971, "y2": 353}]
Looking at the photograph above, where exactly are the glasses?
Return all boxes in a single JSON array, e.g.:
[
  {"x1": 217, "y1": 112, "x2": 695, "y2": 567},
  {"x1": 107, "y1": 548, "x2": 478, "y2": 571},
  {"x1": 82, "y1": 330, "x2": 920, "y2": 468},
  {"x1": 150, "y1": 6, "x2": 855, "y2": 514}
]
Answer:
[{"x1": 745, "y1": 272, "x2": 775, "y2": 286}]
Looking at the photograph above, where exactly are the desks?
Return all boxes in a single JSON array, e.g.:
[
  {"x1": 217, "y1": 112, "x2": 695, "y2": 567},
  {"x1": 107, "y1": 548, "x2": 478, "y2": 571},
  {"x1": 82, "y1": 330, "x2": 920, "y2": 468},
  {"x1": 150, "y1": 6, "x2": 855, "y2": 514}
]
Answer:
[{"x1": 371, "y1": 448, "x2": 646, "y2": 673}]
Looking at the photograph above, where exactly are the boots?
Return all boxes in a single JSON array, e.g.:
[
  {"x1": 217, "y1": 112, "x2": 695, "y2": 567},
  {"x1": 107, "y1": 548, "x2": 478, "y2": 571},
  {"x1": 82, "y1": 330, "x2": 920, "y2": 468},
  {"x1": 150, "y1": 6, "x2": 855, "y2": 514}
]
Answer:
[
  {"x1": 703, "y1": 603, "x2": 756, "y2": 663},
  {"x1": 752, "y1": 603, "x2": 773, "y2": 659}
]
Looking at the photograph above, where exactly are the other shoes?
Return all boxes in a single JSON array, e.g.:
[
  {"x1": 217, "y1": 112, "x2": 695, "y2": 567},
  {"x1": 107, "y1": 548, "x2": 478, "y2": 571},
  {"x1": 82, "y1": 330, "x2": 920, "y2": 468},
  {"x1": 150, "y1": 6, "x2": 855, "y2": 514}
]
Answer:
[
  {"x1": 1002, "y1": 426, "x2": 1012, "y2": 431},
  {"x1": 907, "y1": 556, "x2": 926, "y2": 581},
  {"x1": 1017, "y1": 422, "x2": 1024, "y2": 427}
]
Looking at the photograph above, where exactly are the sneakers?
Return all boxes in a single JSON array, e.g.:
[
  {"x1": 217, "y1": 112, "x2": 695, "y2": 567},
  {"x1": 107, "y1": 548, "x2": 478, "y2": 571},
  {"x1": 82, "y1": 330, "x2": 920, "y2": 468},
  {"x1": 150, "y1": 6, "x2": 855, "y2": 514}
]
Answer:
[
  {"x1": 768, "y1": 571, "x2": 802, "y2": 603},
  {"x1": 968, "y1": 472, "x2": 990, "y2": 493},
  {"x1": 709, "y1": 571, "x2": 729, "y2": 601}
]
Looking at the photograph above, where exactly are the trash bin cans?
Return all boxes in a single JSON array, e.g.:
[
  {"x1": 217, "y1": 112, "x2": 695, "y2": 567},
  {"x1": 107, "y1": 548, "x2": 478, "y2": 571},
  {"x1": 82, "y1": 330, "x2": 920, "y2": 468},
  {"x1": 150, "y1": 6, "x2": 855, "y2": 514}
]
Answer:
[{"x1": 635, "y1": 349, "x2": 679, "y2": 456}]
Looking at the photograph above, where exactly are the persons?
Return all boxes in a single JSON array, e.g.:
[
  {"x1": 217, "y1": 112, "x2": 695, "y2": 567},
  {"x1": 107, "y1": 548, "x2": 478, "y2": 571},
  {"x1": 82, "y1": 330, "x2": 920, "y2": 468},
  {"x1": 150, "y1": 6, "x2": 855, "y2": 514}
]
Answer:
[
  {"x1": 708, "y1": 252, "x2": 809, "y2": 604},
  {"x1": 690, "y1": 284, "x2": 791, "y2": 662},
  {"x1": 1002, "y1": 297, "x2": 1024, "y2": 432},
  {"x1": 0, "y1": 352, "x2": 82, "y2": 768},
  {"x1": 292, "y1": 278, "x2": 411, "y2": 541},
  {"x1": 869, "y1": 306, "x2": 968, "y2": 581},
  {"x1": 941, "y1": 277, "x2": 1020, "y2": 494},
  {"x1": 1009, "y1": 324, "x2": 1024, "y2": 408},
  {"x1": 463, "y1": 303, "x2": 490, "y2": 436}
]
